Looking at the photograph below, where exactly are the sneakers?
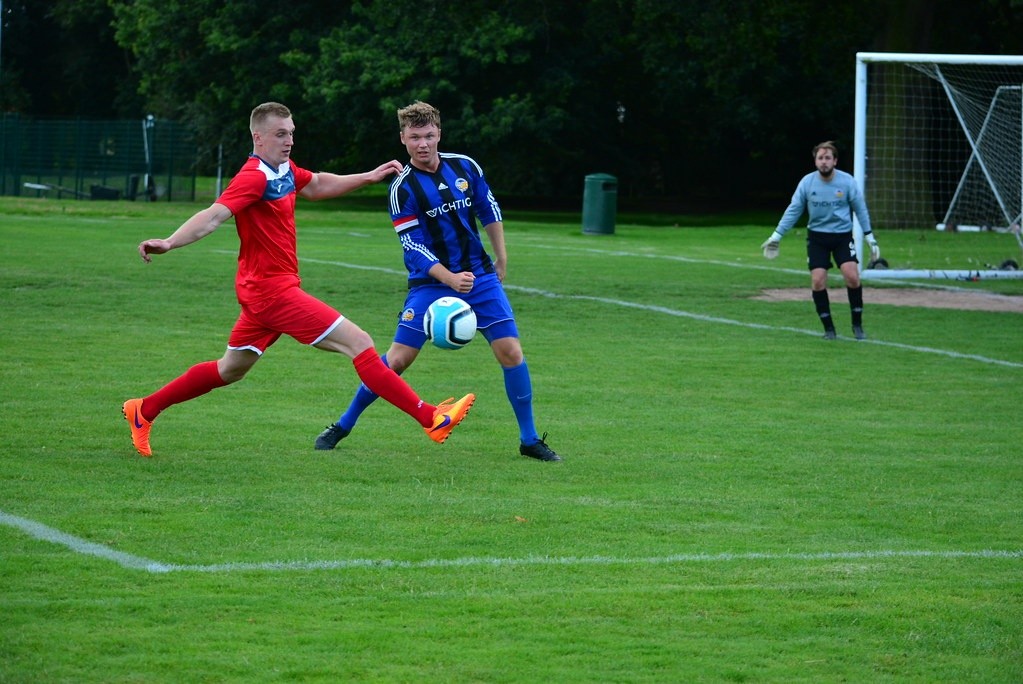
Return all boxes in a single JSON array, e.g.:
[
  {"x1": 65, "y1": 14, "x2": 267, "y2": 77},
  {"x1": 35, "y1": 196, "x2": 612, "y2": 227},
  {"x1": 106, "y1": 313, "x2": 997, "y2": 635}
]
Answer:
[
  {"x1": 520, "y1": 431, "x2": 563, "y2": 462},
  {"x1": 315, "y1": 423, "x2": 346, "y2": 450},
  {"x1": 122, "y1": 399, "x2": 152, "y2": 457},
  {"x1": 424, "y1": 393, "x2": 475, "y2": 443}
]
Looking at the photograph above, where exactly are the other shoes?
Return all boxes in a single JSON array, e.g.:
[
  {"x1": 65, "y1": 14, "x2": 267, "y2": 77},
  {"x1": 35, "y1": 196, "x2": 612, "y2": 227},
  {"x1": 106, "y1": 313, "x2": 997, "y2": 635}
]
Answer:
[
  {"x1": 852, "y1": 326, "x2": 864, "y2": 340},
  {"x1": 825, "y1": 331, "x2": 836, "y2": 341}
]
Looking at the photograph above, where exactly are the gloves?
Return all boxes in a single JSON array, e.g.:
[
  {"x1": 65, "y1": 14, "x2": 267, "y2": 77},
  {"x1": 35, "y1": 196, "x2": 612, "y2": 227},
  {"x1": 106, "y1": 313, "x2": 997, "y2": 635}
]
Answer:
[
  {"x1": 866, "y1": 234, "x2": 880, "y2": 261},
  {"x1": 761, "y1": 230, "x2": 782, "y2": 259}
]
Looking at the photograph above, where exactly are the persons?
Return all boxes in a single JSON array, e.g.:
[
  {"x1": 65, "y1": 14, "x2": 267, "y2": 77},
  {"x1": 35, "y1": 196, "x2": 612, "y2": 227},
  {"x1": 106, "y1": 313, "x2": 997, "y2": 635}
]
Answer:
[
  {"x1": 123, "y1": 103, "x2": 476, "y2": 457},
  {"x1": 761, "y1": 141, "x2": 880, "y2": 340},
  {"x1": 314, "y1": 102, "x2": 562, "y2": 463}
]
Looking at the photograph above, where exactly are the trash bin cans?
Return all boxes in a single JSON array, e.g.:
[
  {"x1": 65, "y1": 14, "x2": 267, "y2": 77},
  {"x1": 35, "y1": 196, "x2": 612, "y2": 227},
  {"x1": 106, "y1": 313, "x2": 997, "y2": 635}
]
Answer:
[
  {"x1": 582, "y1": 172, "x2": 619, "y2": 235},
  {"x1": 91, "y1": 184, "x2": 119, "y2": 200}
]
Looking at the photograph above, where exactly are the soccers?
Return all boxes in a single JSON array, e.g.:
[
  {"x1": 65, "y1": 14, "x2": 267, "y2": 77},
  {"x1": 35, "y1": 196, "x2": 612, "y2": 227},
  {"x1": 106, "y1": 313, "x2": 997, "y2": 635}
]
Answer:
[{"x1": 422, "y1": 296, "x2": 478, "y2": 352}]
